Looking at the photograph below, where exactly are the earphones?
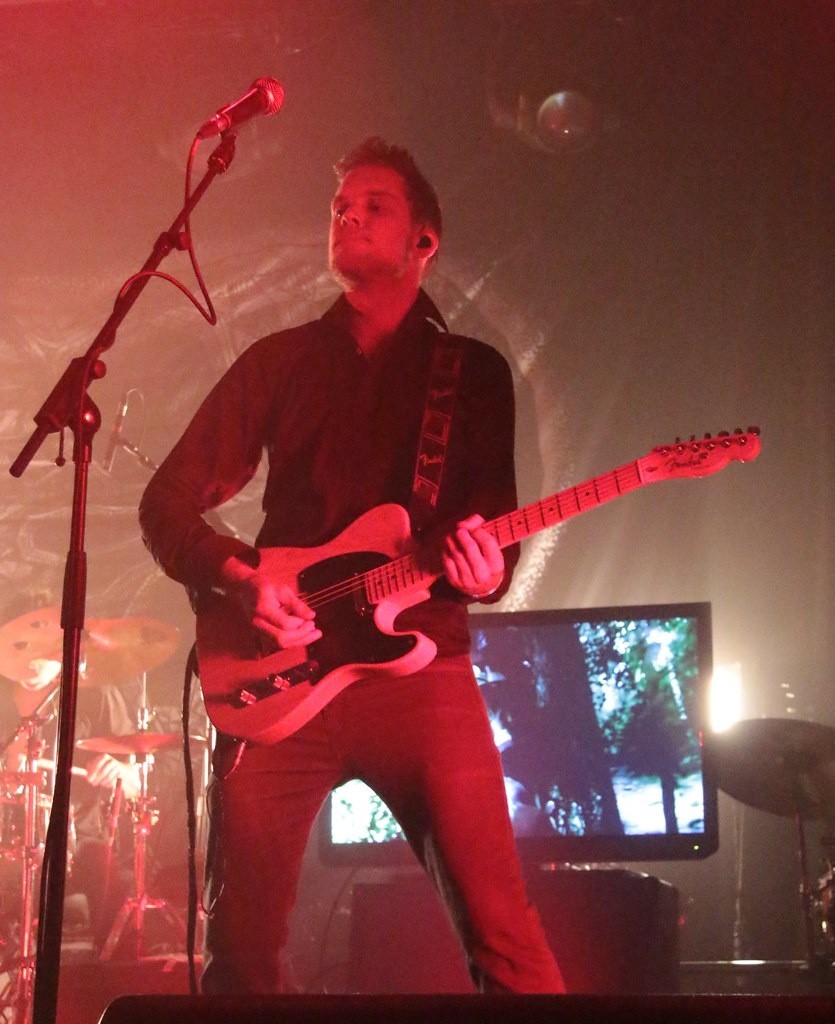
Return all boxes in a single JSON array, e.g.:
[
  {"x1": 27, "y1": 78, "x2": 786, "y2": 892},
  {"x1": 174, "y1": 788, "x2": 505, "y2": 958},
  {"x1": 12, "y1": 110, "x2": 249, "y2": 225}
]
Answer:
[{"x1": 417, "y1": 235, "x2": 431, "y2": 248}]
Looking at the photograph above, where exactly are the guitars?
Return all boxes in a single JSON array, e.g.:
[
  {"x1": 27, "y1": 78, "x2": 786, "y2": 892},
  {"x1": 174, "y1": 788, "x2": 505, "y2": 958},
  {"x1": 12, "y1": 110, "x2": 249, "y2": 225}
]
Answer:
[{"x1": 193, "y1": 420, "x2": 765, "y2": 752}]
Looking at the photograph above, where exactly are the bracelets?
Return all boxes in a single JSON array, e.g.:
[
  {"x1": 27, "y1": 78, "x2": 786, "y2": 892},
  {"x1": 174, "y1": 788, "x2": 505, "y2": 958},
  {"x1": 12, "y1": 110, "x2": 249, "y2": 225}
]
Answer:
[{"x1": 470, "y1": 573, "x2": 505, "y2": 601}]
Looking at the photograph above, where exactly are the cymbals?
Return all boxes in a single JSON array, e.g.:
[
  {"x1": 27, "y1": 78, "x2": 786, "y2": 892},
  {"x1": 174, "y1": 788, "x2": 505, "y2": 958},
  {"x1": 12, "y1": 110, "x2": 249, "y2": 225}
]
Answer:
[
  {"x1": 700, "y1": 716, "x2": 835, "y2": 823},
  {"x1": 73, "y1": 615, "x2": 184, "y2": 692},
  {"x1": 71, "y1": 729, "x2": 186, "y2": 757},
  {"x1": 1, "y1": 602, "x2": 65, "y2": 692}
]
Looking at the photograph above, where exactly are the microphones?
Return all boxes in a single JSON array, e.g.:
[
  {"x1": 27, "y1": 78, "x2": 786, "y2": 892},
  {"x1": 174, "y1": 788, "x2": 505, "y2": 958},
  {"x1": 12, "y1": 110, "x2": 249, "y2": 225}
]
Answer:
[
  {"x1": 102, "y1": 395, "x2": 128, "y2": 471},
  {"x1": 197, "y1": 76, "x2": 285, "y2": 140}
]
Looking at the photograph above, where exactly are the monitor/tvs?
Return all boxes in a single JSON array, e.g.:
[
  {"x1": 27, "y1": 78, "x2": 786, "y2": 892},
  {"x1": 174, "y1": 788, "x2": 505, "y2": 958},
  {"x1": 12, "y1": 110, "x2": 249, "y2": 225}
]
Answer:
[{"x1": 318, "y1": 601, "x2": 722, "y2": 869}]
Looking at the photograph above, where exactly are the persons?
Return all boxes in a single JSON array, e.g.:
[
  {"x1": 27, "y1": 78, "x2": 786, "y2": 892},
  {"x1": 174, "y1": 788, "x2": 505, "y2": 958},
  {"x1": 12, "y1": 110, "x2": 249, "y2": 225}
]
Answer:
[
  {"x1": 1, "y1": 579, "x2": 146, "y2": 954},
  {"x1": 135, "y1": 134, "x2": 573, "y2": 997}
]
